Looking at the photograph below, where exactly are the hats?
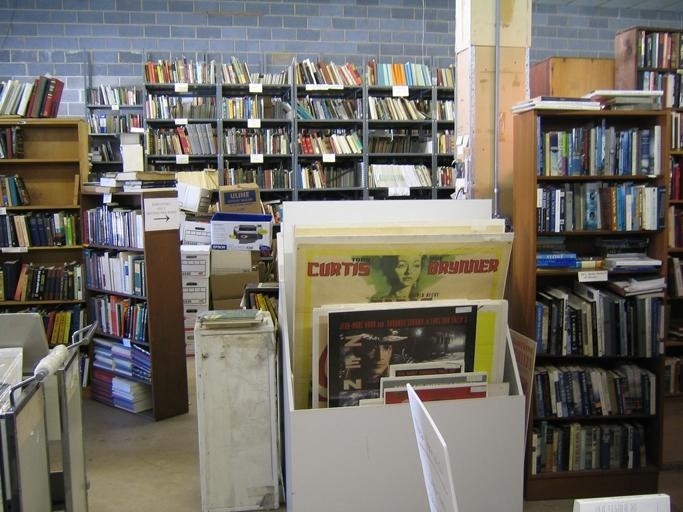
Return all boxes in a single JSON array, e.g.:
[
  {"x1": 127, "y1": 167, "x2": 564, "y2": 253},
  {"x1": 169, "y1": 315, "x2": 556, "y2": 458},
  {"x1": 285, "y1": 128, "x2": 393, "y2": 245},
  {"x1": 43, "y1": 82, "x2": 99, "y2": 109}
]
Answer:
[{"x1": 362, "y1": 328, "x2": 408, "y2": 343}]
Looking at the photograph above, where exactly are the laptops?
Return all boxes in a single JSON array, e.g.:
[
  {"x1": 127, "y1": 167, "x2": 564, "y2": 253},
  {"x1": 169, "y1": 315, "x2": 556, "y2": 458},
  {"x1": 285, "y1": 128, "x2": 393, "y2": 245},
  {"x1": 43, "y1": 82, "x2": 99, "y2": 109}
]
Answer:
[{"x1": 0, "y1": 312, "x2": 50, "y2": 376}]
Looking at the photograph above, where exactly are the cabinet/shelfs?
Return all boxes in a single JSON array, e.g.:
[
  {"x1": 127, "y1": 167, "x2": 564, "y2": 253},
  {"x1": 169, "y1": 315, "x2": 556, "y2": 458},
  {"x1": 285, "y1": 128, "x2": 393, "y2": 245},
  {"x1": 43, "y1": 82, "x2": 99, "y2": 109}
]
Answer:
[{"x1": 1, "y1": 24, "x2": 682, "y2": 502}]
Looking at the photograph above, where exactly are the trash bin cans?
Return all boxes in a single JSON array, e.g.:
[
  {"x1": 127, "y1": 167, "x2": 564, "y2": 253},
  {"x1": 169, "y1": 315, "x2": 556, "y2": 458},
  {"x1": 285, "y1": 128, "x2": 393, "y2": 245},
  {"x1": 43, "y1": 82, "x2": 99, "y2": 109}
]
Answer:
[{"x1": 194, "y1": 309, "x2": 278, "y2": 511}]
